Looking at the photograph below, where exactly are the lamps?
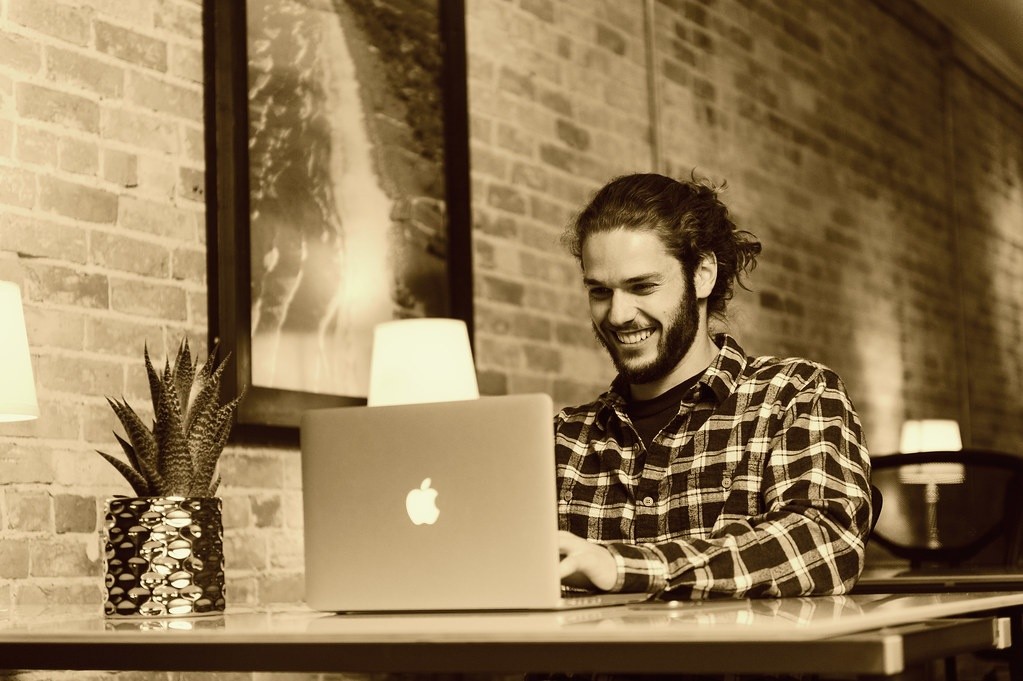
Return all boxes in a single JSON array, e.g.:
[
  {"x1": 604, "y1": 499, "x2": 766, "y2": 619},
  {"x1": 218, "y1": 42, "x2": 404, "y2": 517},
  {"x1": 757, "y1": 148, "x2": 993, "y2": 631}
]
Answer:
[
  {"x1": 898, "y1": 419, "x2": 965, "y2": 549},
  {"x1": 0, "y1": 281, "x2": 41, "y2": 423},
  {"x1": 368, "y1": 318, "x2": 479, "y2": 406}
]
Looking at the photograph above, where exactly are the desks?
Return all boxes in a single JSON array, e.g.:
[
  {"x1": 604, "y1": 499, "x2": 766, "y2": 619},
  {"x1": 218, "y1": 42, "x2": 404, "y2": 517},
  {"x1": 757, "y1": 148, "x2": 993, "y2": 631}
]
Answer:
[{"x1": 0, "y1": 590, "x2": 1023, "y2": 681}]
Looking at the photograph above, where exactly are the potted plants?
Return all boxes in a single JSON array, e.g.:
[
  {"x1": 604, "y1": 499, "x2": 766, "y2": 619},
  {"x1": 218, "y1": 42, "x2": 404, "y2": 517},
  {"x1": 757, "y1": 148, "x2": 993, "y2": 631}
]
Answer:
[{"x1": 92, "y1": 336, "x2": 248, "y2": 620}]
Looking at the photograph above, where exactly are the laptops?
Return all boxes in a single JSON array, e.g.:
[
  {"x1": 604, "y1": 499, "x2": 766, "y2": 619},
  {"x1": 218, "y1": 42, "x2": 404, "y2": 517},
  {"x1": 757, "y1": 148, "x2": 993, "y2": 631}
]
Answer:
[{"x1": 303, "y1": 393, "x2": 651, "y2": 613}]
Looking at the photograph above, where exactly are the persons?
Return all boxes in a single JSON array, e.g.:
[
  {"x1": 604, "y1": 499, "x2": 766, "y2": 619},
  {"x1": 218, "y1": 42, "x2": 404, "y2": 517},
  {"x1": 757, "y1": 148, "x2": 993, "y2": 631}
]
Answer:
[{"x1": 553, "y1": 172, "x2": 872, "y2": 600}]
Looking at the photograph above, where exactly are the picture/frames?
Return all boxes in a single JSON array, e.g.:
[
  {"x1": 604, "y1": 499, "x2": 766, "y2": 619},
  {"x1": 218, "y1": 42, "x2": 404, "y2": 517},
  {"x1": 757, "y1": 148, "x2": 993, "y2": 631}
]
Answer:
[{"x1": 203, "y1": 0, "x2": 474, "y2": 450}]
